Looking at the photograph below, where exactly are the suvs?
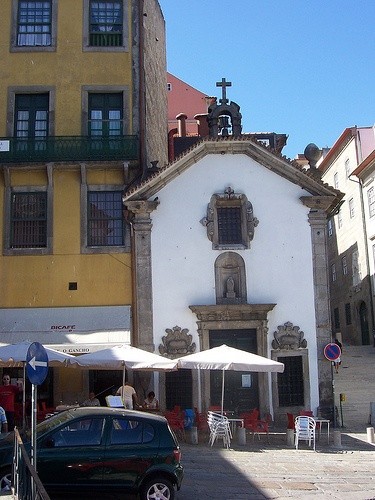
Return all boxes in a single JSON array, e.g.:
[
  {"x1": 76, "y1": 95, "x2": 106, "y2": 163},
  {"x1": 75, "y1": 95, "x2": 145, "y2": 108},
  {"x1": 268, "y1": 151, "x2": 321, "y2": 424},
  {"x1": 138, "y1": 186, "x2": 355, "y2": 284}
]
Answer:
[{"x1": 0, "y1": 405, "x2": 185, "y2": 500}]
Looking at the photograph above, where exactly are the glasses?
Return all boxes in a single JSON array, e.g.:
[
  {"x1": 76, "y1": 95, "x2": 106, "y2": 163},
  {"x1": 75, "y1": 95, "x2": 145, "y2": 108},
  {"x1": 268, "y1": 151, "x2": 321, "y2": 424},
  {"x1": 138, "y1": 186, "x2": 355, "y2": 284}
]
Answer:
[{"x1": 3, "y1": 377, "x2": 10, "y2": 381}]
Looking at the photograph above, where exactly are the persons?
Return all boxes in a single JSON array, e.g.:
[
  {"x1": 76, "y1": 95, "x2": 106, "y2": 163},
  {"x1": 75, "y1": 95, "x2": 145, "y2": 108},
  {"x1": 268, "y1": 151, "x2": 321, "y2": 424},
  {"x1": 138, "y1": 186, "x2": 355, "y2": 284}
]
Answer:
[
  {"x1": 67, "y1": 345, "x2": 177, "y2": 407},
  {"x1": 0, "y1": 374, "x2": 17, "y2": 431},
  {"x1": 225, "y1": 275, "x2": 235, "y2": 293},
  {"x1": 143, "y1": 391, "x2": 159, "y2": 410},
  {"x1": 0, "y1": 406, "x2": 8, "y2": 434},
  {"x1": 82, "y1": 391, "x2": 100, "y2": 407},
  {"x1": 115, "y1": 381, "x2": 138, "y2": 410},
  {"x1": 333, "y1": 338, "x2": 343, "y2": 375}
]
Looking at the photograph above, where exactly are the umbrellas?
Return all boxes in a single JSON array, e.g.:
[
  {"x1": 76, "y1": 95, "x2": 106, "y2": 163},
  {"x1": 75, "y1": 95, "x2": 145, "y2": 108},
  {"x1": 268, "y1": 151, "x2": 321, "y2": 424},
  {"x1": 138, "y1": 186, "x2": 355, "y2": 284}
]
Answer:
[
  {"x1": 171, "y1": 345, "x2": 285, "y2": 420},
  {"x1": 0, "y1": 339, "x2": 75, "y2": 430}
]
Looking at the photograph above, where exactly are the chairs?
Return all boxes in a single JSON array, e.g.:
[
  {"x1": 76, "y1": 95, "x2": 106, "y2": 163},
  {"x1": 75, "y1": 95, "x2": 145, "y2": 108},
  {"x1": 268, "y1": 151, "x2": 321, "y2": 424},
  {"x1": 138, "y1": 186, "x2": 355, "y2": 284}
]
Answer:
[
  {"x1": 240, "y1": 407, "x2": 273, "y2": 442},
  {"x1": 286, "y1": 408, "x2": 320, "y2": 450},
  {"x1": 13, "y1": 399, "x2": 54, "y2": 427},
  {"x1": 160, "y1": 404, "x2": 234, "y2": 450}
]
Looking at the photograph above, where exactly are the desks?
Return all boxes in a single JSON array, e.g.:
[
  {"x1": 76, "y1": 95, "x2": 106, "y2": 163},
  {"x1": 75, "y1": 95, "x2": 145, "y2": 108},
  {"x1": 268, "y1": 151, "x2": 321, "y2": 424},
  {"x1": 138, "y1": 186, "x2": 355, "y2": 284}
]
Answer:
[
  {"x1": 306, "y1": 416, "x2": 331, "y2": 446},
  {"x1": 209, "y1": 409, "x2": 234, "y2": 437},
  {"x1": 56, "y1": 405, "x2": 79, "y2": 410}
]
